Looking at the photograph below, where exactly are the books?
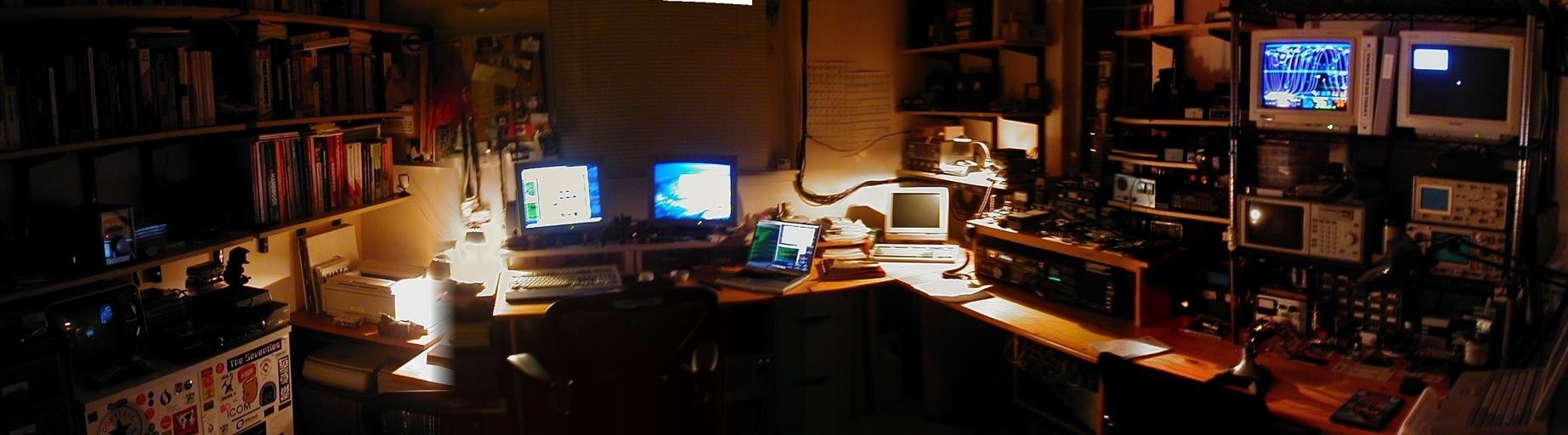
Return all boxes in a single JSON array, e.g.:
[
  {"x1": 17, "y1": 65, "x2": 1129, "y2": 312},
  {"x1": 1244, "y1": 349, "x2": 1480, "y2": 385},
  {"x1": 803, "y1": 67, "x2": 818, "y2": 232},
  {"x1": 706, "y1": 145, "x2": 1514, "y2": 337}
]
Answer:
[
  {"x1": 297, "y1": 225, "x2": 491, "y2": 396},
  {"x1": 816, "y1": 217, "x2": 886, "y2": 280},
  {"x1": 132, "y1": 21, "x2": 415, "y2": 223}
]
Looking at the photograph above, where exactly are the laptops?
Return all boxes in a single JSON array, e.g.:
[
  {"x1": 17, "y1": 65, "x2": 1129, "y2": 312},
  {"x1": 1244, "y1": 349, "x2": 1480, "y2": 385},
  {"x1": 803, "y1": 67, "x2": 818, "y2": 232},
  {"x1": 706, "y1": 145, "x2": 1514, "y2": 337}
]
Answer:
[{"x1": 715, "y1": 217, "x2": 823, "y2": 294}]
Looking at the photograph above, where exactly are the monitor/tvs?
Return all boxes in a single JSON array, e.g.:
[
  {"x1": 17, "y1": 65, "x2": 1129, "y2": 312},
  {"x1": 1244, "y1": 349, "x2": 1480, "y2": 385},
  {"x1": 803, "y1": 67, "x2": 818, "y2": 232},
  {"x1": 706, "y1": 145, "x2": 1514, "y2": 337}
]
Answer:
[
  {"x1": 1396, "y1": 30, "x2": 1524, "y2": 146},
  {"x1": 515, "y1": 157, "x2": 604, "y2": 245},
  {"x1": 649, "y1": 155, "x2": 736, "y2": 240},
  {"x1": 884, "y1": 187, "x2": 949, "y2": 241},
  {"x1": 1249, "y1": 28, "x2": 1364, "y2": 133}
]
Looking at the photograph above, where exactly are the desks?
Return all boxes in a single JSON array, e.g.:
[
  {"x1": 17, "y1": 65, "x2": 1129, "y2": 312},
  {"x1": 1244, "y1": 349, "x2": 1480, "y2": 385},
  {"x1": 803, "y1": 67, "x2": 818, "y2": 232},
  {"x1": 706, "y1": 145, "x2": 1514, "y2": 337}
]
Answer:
[
  {"x1": 296, "y1": 241, "x2": 893, "y2": 432},
  {"x1": 896, "y1": 238, "x2": 1551, "y2": 434}
]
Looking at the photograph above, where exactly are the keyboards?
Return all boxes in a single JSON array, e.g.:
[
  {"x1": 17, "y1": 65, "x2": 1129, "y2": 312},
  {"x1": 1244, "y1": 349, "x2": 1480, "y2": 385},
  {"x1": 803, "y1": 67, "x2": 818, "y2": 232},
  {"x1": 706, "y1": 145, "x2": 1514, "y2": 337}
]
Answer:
[
  {"x1": 868, "y1": 244, "x2": 960, "y2": 262},
  {"x1": 506, "y1": 264, "x2": 625, "y2": 301}
]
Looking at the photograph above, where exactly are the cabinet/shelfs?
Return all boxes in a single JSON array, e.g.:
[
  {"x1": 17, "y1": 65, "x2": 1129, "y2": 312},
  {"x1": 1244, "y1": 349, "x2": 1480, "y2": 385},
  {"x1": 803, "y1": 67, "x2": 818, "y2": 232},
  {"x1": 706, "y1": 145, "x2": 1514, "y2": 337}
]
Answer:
[
  {"x1": 0, "y1": 5, "x2": 416, "y2": 308},
  {"x1": 893, "y1": 37, "x2": 1052, "y2": 191},
  {"x1": 1105, "y1": 17, "x2": 1253, "y2": 225}
]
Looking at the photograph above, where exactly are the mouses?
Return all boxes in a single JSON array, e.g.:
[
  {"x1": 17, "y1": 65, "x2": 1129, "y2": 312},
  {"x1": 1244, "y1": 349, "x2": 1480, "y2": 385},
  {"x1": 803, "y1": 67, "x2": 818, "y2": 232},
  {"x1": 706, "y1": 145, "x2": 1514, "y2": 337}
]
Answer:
[
  {"x1": 638, "y1": 270, "x2": 654, "y2": 282},
  {"x1": 670, "y1": 269, "x2": 690, "y2": 283}
]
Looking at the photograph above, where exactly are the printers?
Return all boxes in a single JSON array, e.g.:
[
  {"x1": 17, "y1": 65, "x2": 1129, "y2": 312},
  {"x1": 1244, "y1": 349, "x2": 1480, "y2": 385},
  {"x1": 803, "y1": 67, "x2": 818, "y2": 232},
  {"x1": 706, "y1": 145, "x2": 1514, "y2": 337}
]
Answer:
[{"x1": 321, "y1": 259, "x2": 432, "y2": 324}]
